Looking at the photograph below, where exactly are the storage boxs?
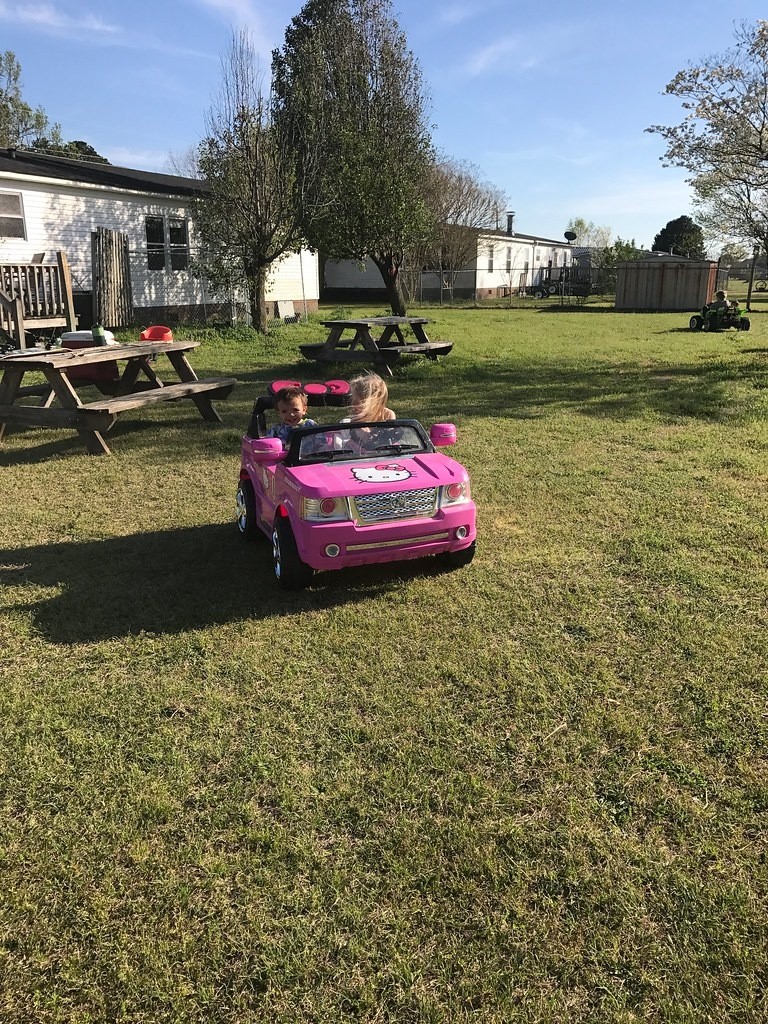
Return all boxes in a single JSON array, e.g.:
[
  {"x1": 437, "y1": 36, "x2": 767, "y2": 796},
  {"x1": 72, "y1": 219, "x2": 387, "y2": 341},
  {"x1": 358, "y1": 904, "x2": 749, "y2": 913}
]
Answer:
[{"x1": 60, "y1": 330, "x2": 115, "y2": 378}]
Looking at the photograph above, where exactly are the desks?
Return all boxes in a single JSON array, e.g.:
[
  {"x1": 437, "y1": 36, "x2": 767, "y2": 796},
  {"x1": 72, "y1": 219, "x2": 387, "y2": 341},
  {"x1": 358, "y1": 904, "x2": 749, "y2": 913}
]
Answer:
[
  {"x1": 319, "y1": 319, "x2": 428, "y2": 377},
  {"x1": 0, "y1": 341, "x2": 222, "y2": 454}
]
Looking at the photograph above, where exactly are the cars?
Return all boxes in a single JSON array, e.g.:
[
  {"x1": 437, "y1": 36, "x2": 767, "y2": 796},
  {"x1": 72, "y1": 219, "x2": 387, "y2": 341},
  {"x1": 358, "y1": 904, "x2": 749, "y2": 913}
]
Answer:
[
  {"x1": 235, "y1": 379, "x2": 476, "y2": 591},
  {"x1": 690, "y1": 299, "x2": 751, "y2": 333}
]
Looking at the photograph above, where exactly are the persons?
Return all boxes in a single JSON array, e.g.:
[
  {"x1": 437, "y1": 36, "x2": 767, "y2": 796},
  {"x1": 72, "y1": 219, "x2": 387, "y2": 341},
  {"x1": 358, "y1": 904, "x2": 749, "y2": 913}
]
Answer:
[
  {"x1": 702, "y1": 290, "x2": 739, "y2": 318},
  {"x1": 267, "y1": 387, "x2": 327, "y2": 456},
  {"x1": 334, "y1": 369, "x2": 400, "y2": 454}
]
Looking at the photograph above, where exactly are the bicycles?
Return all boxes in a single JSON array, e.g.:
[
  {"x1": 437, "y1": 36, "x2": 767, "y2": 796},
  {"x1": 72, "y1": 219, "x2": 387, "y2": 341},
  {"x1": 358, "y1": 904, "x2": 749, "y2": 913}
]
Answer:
[{"x1": 756, "y1": 274, "x2": 768, "y2": 292}]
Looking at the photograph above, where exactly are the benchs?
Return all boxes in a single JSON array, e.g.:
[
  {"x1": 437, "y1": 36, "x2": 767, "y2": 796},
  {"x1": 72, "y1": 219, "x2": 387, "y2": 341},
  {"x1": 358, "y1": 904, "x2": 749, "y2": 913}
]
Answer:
[
  {"x1": 297, "y1": 339, "x2": 360, "y2": 356},
  {"x1": 379, "y1": 341, "x2": 453, "y2": 354},
  {"x1": 77, "y1": 377, "x2": 237, "y2": 409}
]
